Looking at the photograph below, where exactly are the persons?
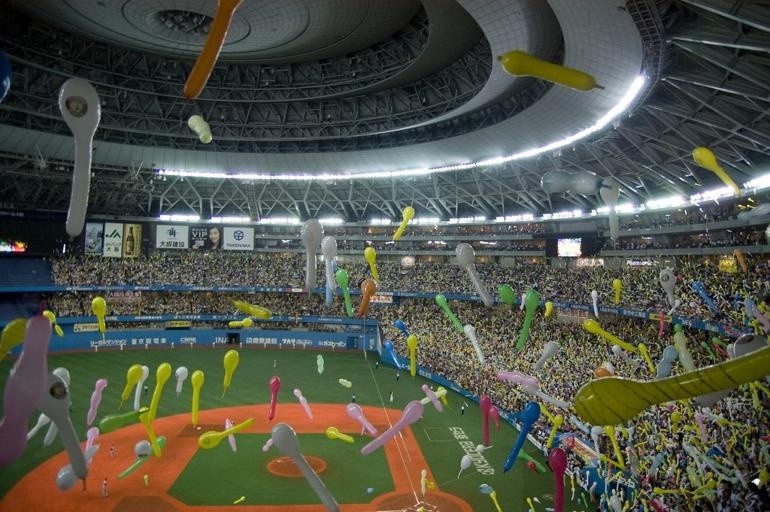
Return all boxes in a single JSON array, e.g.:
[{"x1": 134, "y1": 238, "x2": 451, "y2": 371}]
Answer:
[{"x1": 47, "y1": 201, "x2": 770, "y2": 512}]
